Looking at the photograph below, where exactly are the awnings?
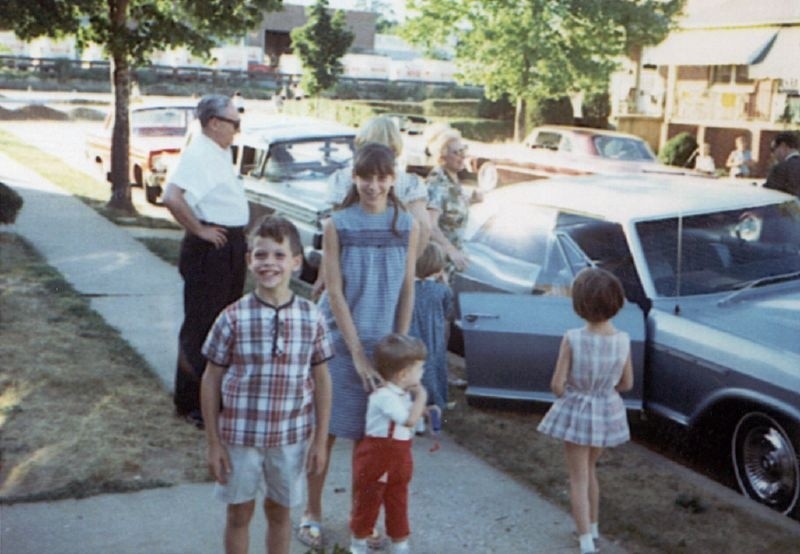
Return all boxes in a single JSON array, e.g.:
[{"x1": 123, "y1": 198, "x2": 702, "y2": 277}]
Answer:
[{"x1": 643, "y1": 27, "x2": 785, "y2": 69}]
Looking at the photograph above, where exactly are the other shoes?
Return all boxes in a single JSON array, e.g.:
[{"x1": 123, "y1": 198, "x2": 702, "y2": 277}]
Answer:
[
  {"x1": 295, "y1": 515, "x2": 322, "y2": 550},
  {"x1": 351, "y1": 538, "x2": 366, "y2": 553},
  {"x1": 390, "y1": 541, "x2": 411, "y2": 554},
  {"x1": 571, "y1": 530, "x2": 600, "y2": 552},
  {"x1": 366, "y1": 528, "x2": 386, "y2": 548},
  {"x1": 174, "y1": 407, "x2": 205, "y2": 429}
]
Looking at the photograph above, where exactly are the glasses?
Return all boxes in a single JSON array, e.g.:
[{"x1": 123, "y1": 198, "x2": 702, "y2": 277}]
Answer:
[
  {"x1": 452, "y1": 144, "x2": 468, "y2": 156},
  {"x1": 209, "y1": 116, "x2": 240, "y2": 130}
]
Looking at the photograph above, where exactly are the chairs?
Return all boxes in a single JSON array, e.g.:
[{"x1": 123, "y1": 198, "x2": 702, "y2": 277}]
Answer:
[
  {"x1": 264, "y1": 148, "x2": 292, "y2": 175},
  {"x1": 615, "y1": 258, "x2": 673, "y2": 286},
  {"x1": 706, "y1": 244, "x2": 732, "y2": 266}
]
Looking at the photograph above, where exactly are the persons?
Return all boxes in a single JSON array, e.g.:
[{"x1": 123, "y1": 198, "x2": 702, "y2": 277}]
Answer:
[
  {"x1": 697, "y1": 132, "x2": 800, "y2": 249},
  {"x1": 162, "y1": 73, "x2": 474, "y2": 554},
  {"x1": 535, "y1": 270, "x2": 634, "y2": 554}
]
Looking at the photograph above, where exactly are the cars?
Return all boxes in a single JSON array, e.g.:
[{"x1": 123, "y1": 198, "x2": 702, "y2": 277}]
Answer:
[
  {"x1": 92, "y1": 98, "x2": 206, "y2": 204},
  {"x1": 451, "y1": 171, "x2": 800, "y2": 516},
  {"x1": 223, "y1": 116, "x2": 692, "y2": 283}
]
